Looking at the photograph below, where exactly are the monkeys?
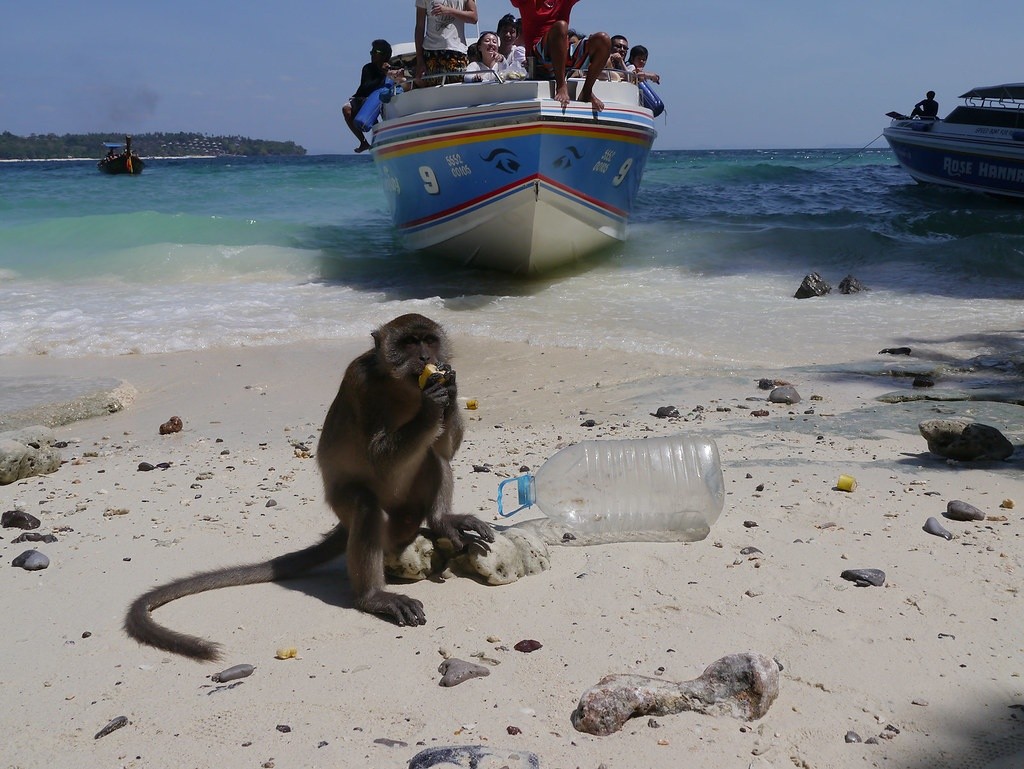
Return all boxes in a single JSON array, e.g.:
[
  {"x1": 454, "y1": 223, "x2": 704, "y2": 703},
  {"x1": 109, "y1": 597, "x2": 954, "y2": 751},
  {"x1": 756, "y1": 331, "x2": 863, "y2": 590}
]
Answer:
[{"x1": 124, "y1": 313, "x2": 495, "y2": 663}]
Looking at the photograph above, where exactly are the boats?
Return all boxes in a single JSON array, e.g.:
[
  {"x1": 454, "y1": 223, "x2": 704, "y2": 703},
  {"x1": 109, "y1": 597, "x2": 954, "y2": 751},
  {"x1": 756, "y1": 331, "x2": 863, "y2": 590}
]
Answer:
[
  {"x1": 371, "y1": 36, "x2": 658, "y2": 281},
  {"x1": 880, "y1": 81, "x2": 1024, "y2": 210},
  {"x1": 97, "y1": 135, "x2": 146, "y2": 175}
]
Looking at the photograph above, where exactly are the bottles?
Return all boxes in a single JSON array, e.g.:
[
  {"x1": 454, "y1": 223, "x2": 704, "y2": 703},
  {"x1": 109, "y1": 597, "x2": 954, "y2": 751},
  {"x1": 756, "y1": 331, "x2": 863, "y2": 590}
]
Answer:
[{"x1": 497, "y1": 434, "x2": 725, "y2": 535}]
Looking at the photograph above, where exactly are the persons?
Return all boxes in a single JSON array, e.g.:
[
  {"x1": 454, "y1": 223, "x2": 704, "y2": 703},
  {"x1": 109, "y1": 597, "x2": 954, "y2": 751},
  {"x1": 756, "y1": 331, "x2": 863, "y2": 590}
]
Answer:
[
  {"x1": 910, "y1": 91, "x2": 938, "y2": 119},
  {"x1": 341, "y1": 0, "x2": 661, "y2": 154}
]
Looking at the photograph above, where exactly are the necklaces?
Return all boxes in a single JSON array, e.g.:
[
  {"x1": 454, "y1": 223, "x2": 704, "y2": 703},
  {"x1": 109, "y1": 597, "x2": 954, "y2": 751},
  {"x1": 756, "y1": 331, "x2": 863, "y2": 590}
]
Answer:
[{"x1": 544, "y1": 2, "x2": 555, "y2": 8}]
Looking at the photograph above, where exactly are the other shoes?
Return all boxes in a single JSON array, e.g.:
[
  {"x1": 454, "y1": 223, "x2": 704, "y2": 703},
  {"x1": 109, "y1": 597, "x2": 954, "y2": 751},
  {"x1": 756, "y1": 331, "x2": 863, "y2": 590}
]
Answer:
[{"x1": 354, "y1": 142, "x2": 370, "y2": 153}]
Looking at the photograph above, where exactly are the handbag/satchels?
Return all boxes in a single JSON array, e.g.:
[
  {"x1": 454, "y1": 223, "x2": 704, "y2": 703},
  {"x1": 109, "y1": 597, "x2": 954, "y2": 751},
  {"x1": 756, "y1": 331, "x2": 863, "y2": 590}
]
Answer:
[{"x1": 639, "y1": 79, "x2": 664, "y2": 117}]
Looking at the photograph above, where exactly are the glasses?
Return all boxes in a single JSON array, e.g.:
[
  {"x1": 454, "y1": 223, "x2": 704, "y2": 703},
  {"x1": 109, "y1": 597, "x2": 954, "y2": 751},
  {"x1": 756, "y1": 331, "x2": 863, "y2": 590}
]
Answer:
[
  {"x1": 370, "y1": 50, "x2": 381, "y2": 55},
  {"x1": 502, "y1": 17, "x2": 519, "y2": 23}
]
